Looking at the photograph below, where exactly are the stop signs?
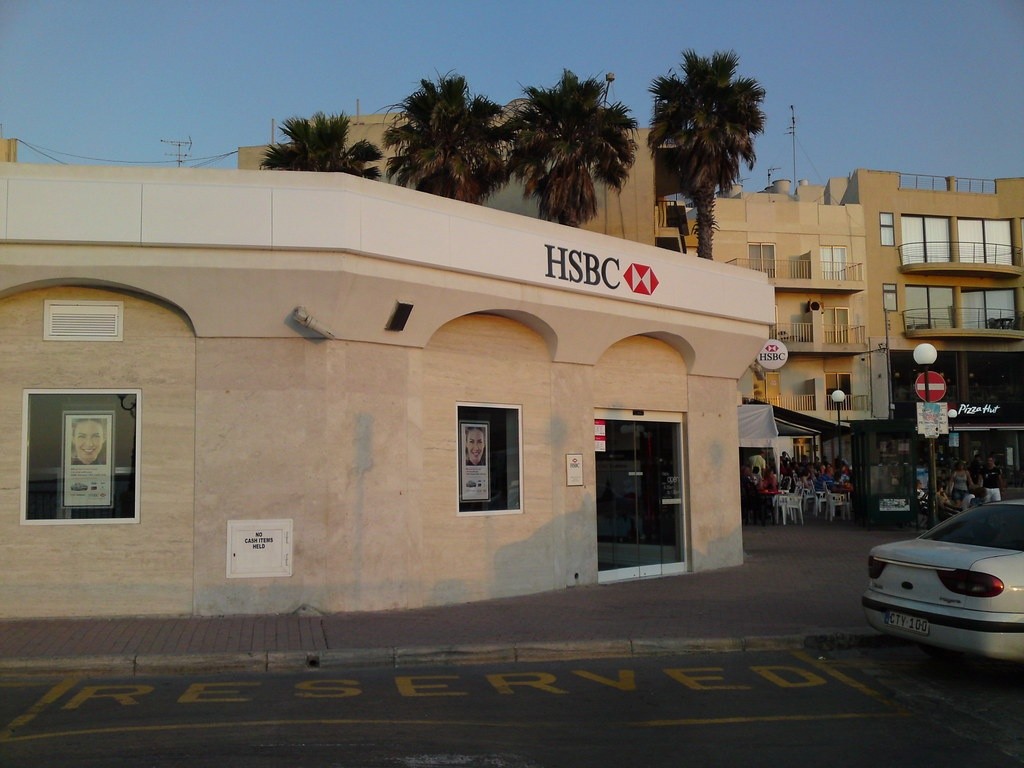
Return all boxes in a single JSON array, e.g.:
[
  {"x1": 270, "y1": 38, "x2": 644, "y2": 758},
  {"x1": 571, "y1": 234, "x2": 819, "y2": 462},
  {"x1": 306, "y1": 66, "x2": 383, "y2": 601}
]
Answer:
[{"x1": 916, "y1": 371, "x2": 947, "y2": 403}]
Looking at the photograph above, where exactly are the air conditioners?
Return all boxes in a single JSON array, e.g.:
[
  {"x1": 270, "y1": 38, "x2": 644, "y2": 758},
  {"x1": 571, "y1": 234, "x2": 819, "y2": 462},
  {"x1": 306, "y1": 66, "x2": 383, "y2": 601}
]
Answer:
[{"x1": 807, "y1": 300, "x2": 825, "y2": 314}]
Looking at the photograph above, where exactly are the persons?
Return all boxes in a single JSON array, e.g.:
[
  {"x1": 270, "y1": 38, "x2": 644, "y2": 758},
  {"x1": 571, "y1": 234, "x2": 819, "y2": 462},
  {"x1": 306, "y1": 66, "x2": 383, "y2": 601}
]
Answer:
[
  {"x1": 917, "y1": 452, "x2": 1005, "y2": 516},
  {"x1": 742, "y1": 450, "x2": 852, "y2": 522},
  {"x1": 72, "y1": 418, "x2": 106, "y2": 464},
  {"x1": 464, "y1": 428, "x2": 485, "y2": 466}
]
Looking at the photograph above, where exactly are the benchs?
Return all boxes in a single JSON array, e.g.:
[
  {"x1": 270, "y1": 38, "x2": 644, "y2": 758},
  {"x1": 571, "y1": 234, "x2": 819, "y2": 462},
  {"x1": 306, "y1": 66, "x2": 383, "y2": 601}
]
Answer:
[{"x1": 921, "y1": 498, "x2": 963, "y2": 530}]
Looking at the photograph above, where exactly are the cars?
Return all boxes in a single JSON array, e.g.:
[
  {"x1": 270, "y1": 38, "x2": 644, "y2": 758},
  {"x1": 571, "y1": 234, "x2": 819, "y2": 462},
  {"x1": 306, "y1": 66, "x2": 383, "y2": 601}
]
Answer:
[{"x1": 863, "y1": 498, "x2": 1024, "y2": 663}]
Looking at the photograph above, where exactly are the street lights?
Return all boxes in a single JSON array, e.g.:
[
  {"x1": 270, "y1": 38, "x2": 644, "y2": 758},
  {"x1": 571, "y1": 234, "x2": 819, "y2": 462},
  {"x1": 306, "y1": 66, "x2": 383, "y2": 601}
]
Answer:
[
  {"x1": 831, "y1": 388, "x2": 846, "y2": 476},
  {"x1": 914, "y1": 343, "x2": 938, "y2": 530}
]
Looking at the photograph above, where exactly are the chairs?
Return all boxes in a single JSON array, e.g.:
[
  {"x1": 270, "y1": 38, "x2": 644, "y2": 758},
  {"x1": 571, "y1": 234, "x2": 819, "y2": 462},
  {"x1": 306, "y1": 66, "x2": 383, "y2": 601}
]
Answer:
[
  {"x1": 778, "y1": 483, "x2": 804, "y2": 525},
  {"x1": 825, "y1": 482, "x2": 851, "y2": 522},
  {"x1": 793, "y1": 477, "x2": 817, "y2": 516},
  {"x1": 772, "y1": 481, "x2": 802, "y2": 521},
  {"x1": 782, "y1": 476, "x2": 792, "y2": 491},
  {"x1": 810, "y1": 480, "x2": 826, "y2": 518}
]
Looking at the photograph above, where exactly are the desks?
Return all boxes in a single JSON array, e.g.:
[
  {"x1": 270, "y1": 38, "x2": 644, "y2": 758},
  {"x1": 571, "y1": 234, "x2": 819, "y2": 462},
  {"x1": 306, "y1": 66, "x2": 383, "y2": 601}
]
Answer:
[
  {"x1": 995, "y1": 317, "x2": 1013, "y2": 330},
  {"x1": 751, "y1": 489, "x2": 782, "y2": 527}
]
